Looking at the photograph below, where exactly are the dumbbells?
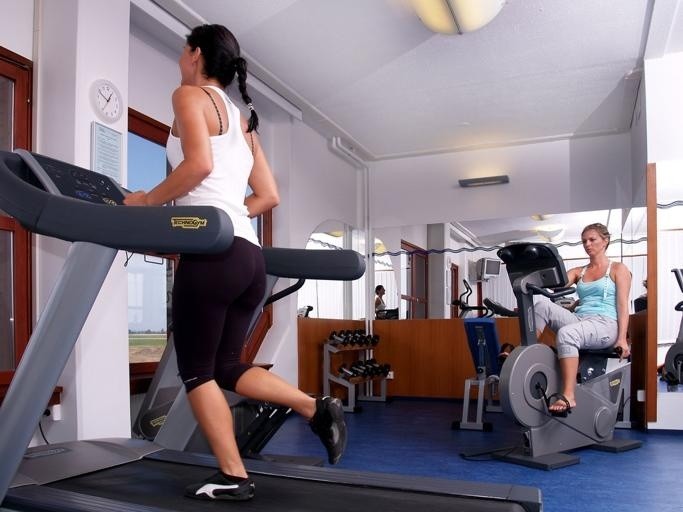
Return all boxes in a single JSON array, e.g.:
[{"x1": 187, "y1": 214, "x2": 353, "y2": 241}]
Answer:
[
  {"x1": 329, "y1": 329, "x2": 380, "y2": 346},
  {"x1": 338, "y1": 358, "x2": 390, "y2": 378}
]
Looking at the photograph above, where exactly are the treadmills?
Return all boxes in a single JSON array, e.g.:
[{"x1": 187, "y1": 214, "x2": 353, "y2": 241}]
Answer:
[{"x1": 1, "y1": 147, "x2": 543, "y2": 511}]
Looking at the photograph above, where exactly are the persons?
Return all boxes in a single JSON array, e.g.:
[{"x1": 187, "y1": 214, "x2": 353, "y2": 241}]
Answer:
[
  {"x1": 630, "y1": 277, "x2": 648, "y2": 314},
  {"x1": 114, "y1": 22, "x2": 350, "y2": 503},
  {"x1": 494, "y1": 223, "x2": 633, "y2": 414},
  {"x1": 373, "y1": 285, "x2": 387, "y2": 312}
]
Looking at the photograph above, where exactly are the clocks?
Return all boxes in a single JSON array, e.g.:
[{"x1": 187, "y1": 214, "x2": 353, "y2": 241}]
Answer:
[{"x1": 87, "y1": 77, "x2": 124, "y2": 123}]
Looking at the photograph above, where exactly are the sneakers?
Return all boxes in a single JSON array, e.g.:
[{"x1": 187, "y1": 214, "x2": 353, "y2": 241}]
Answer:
[
  {"x1": 307, "y1": 396, "x2": 348, "y2": 466},
  {"x1": 184, "y1": 470, "x2": 255, "y2": 500}
]
